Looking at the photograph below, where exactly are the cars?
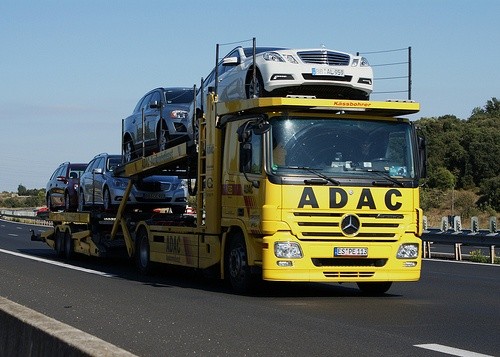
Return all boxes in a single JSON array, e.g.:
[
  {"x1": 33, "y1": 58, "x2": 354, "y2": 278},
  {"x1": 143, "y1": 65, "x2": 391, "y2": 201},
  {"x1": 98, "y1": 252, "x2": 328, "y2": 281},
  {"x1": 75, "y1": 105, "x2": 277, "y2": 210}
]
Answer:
[
  {"x1": 45, "y1": 161, "x2": 90, "y2": 213},
  {"x1": 122, "y1": 87, "x2": 201, "y2": 163},
  {"x1": 78, "y1": 152, "x2": 187, "y2": 217},
  {"x1": 188, "y1": 46, "x2": 374, "y2": 139}
]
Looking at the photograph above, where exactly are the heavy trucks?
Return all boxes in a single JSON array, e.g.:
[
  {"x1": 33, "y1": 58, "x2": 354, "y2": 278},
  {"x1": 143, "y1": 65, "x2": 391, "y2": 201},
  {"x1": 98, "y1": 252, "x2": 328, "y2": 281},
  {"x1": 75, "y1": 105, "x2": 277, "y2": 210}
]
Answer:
[{"x1": 30, "y1": 90, "x2": 427, "y2": 298}]
